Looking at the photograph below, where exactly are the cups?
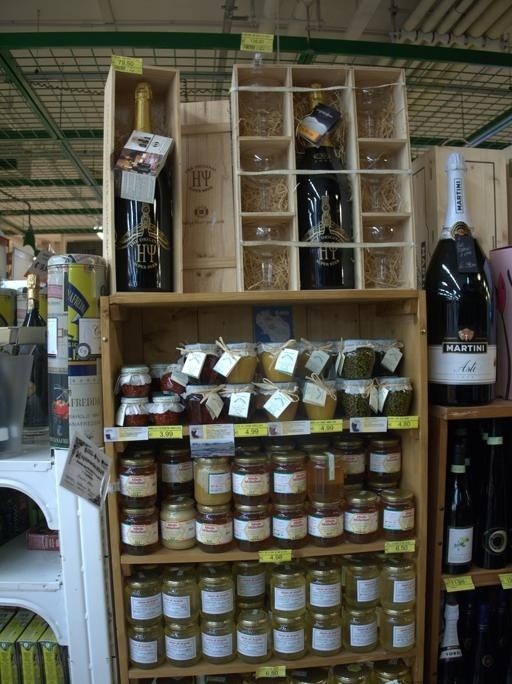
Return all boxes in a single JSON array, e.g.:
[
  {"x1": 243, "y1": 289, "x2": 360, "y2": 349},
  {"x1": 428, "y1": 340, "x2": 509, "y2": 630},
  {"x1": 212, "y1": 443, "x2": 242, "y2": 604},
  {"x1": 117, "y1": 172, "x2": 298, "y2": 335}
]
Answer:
[
  {"x1": 240, "y1": 77, "x2": 281, "y2": 136},
  {"x1": 359, "y1": 149, "x2": 392, "y2": 213},
  {"x1": 242, "y1": 146, "x2": 283, "y2": 212},
  {"x1": 242, "y1": 221, "x2": 287, "y2": 291},
  {"x1": 0, "y1": 355, "x2": 32, "y2": 460},
  {"x1": 364, "y1": 225, "x2": 394, "y2": 288},
  {"x1": 357, "y1": 80, "x2": 392, "y2": 138}
]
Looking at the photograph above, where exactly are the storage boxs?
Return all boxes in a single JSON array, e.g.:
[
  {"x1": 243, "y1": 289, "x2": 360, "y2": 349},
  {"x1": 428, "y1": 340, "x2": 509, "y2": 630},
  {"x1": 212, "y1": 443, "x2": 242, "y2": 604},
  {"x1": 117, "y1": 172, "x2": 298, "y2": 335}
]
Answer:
[
  {"x1": 230, "y1": 66, "x2": 417, "y2": 290},
  {"x1": 412, "y1": 146, "x2": 509, "y2": 289},
  {"x1": 103, "y1": 63, "x2": 183, "y2": 294}
]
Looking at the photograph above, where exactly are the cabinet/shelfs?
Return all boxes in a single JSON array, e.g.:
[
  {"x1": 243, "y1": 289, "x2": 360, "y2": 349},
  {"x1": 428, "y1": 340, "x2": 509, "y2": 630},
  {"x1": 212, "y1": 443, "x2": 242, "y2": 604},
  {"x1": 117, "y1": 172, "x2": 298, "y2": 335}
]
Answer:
[
  {"x1": 428, "y1": 401, "x2": 512, "y2": 684},
  {"x1": 98, "y1": 290, "x2": 426, "y2": 683}
]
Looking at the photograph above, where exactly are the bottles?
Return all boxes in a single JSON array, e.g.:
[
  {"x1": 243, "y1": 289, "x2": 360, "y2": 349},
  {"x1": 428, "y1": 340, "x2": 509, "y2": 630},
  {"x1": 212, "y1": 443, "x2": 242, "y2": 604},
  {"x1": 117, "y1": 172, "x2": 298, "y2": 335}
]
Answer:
[
  {"x1": 307, "y1": 610, "x2": 342, "y2": 656},
  {"x1": 200, "y1": 619, "x2": 236, "y2": 665},
  {"x1": 333, "y1": 664, "x2": 369, "y2": 684},
  {"x1": 367, "y1": 438, "x2": 401, "y2": 482},
  {"x1": 195, "y1": 504, "x2": 233, "y2": 554},
  {"x1": 438, "y1": 602, "x2": 465, "y2": 684},
  {"x1": 474, "y1": 426, "x2": 512, "y2": 569},
  {"x1": 342, "y1": 608, "x2": 378, "y2": 654},
  {"x1": 198, "y1": 566, "x2": 235, "y2": 620},
  {"x1": 159, "y1": 497, "x2": 195, "y2": 550},
  {"x1": 441, "y1": 450, "x2": 474, "y2": 575},
  {"x1": 162, "y1": 571, "x2": 199, "y2": 624},
  {"x1": 233, "y1": 503, "x2": 271, "y2": 552},
  {"x1": 123, "y1": 573, "x2": 161, "y2": 627},
  {"x1": 118, "y1": 457, "x2": 158, "y2": 509},
  {"x1": 372, "y1": 658, "x2": 413, "y2": 684},
  {"x1": 272, "y1": 504, "x2": 308, "y2": 550},
  {"x1": 368, "y1": 481, "x2": 397, "y2": 496},
  {"x1": 332, "y1": 439, "x2": 366, "y2": 486},
  {"x1": 233, "y1": 441, "x2": 330, "y2": 455},
  {"x1": 163, "y1": 624, "x2": 201, "y2": 668},
  {"x1": 270, "y1": 614, "x2": 307, "y2": 661},
  {"x1": 269, "y1": 564, "x2": 306, "y2": 618},
  {"x1": 235, "y1": 609, "x2": 272, "y2": 664},
  {"x1": 379, "y1": 609, "x2": 415, "y2": 653},
  {"x1": 235, "y1": 560, "x2": 265, "y2": 601},
  {"x1": 14, "y1": 273, "x2": 48, "y2": 429},
  {"x1": 127, "y1": 625, "x2": 165, "y2": 669},
  {"x1": 307, "y1": 502, "x2": 343, "y2": 547},
  {"x1": 298, "y1": 82, "x2": 347, "y2": 289},
  {"x1": 307, "y1": 452, "x2": 344, "y2": 503},
  {"x1": 345, "y1": 560, "x2": 380, "y2": 607},
  {"x1": 194, "y1": 456, "x2": 232, "y2": 505},
  {"x1": 306, "y1": 561, "x2": 342, "y2": 612},
  {"x1": 237, "y1": 602, "x2": 266, "y2": 611},
  {"x1": 380, "y1": 559, "x2": 416, "y2": 610},
  {"x1": 270, "y1": 453, "x2": 307, "y2": 505},
  {"x1": 114, "y1": 82, "x2": 171, "y2": 292},
  {"x1": 380, "y1": 488, "x2": 415, "y2": 541},
  {"x1": 343, "y1": 490, "x2": 380, "y2": 544},
  {"x1": 159, "y1": 448, "x2": 192, "y2": 495},
  {"x1": 120, "y1": 506, "x2": 159, "y2": 556},
  {"x1": 426, "y1": 152, "x2": 497, "y2": 406},
  {"x1": 232, "y1": 456, "x2": 270, "y2": 506}
]
